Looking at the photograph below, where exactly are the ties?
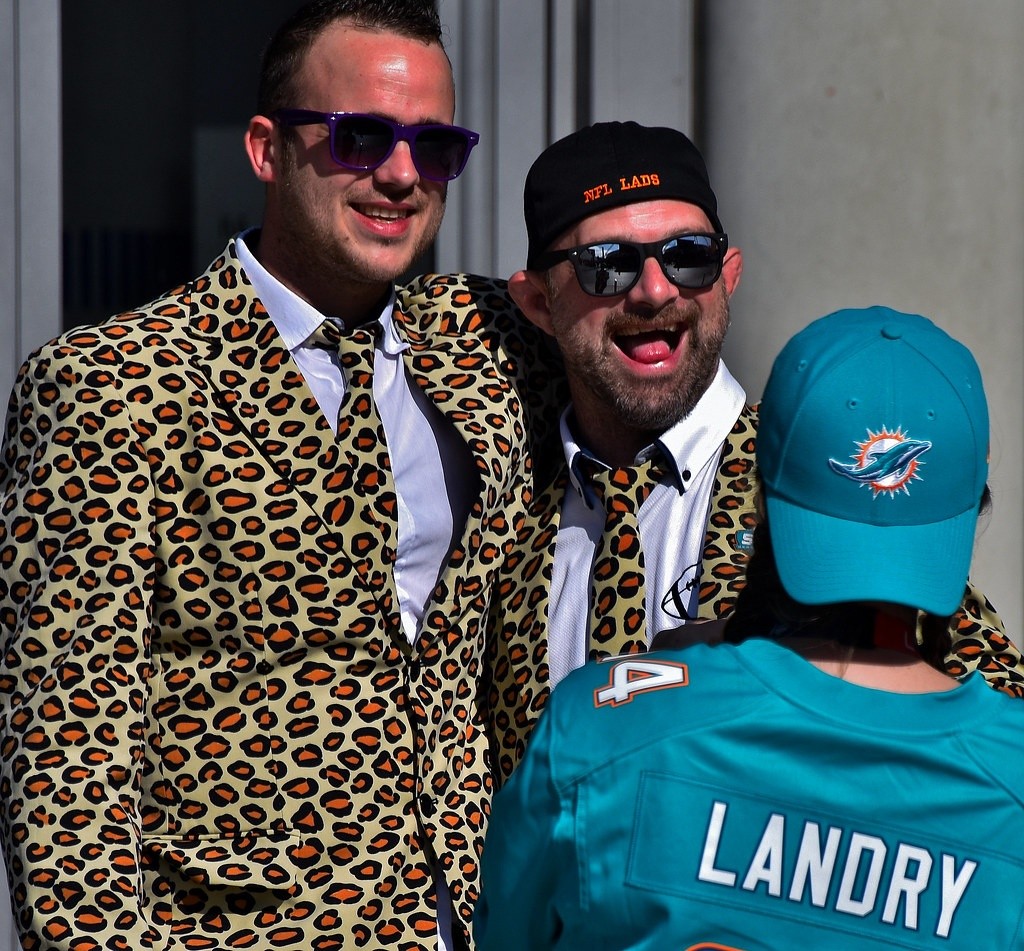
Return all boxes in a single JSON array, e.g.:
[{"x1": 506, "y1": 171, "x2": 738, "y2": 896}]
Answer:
[
  {"x1": 303, "y1": 319, "x2": 398, "y2": 576},
  {"x1": 575, "y1": 450, "x2": 672, "y2": 662}
]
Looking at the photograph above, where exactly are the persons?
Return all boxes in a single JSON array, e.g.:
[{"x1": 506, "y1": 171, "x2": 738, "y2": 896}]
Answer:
[
  {"x1": 474, "y1": 306, "x2": 1024, "y2": 951},
  {"x1": 0, "y1": 0, "x2": 569, "y2": 951},
  {"x1": 483, "y1": 120, "x2": 1024, "y2": 795}
]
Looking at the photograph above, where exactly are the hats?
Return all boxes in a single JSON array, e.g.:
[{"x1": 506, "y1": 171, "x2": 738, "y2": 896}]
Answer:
[
  {"x1": 754, "y1": 305, "x2": 990, "y2": 616},
  {"x1": 523, "y1": 121, "x2": 723, "y2": 270}
]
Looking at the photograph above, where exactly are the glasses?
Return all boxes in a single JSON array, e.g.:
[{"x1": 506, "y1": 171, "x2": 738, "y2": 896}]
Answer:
[
  {"x1": 541, "y1": 230, "x2": 729, "y2": 297},
  {"x1": 271, "y1": 106, "x2": 480, "y2": 181}
]
